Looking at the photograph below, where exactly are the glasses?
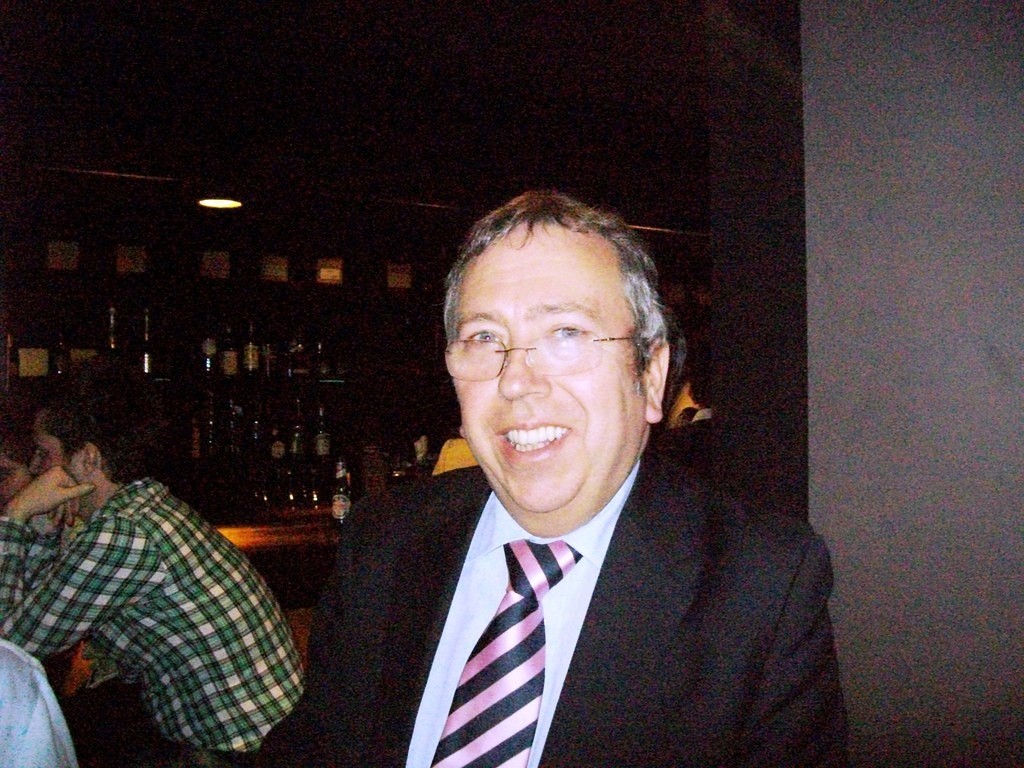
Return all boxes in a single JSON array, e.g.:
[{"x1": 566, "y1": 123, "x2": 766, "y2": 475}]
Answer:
[{"x1": 443, "y1": 331, "x2": 639, "y2": 382}]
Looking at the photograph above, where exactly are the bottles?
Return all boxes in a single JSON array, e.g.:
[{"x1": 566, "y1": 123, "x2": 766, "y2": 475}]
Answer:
[
  {"x1": 50, "y1": 300, "x2": 330, "y2": 503},
  {"x1": 331, "y1": 459, "x2": 351, "y2": 529}
]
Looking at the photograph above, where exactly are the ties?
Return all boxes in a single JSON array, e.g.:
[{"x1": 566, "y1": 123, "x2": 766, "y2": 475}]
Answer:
[{"x1": 430, "y1": 538, "x2": 583, "y2": 768}]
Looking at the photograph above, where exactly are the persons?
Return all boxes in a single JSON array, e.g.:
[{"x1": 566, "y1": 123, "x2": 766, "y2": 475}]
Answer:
[
  {"x1": 253, "y1": 189, "x2": 853, "y2": 768},
  {"x1": 0, "y1": 378, "x2": 304, "y2": 768}
]
